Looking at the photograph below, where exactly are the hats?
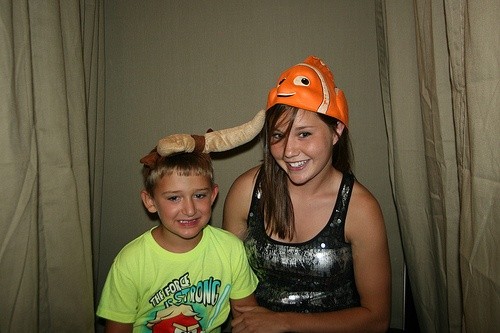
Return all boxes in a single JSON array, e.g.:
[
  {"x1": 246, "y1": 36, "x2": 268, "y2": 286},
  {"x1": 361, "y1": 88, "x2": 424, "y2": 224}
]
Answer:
[{"x1": 265, "y1": 55, "x2": 350, "y2": 135}]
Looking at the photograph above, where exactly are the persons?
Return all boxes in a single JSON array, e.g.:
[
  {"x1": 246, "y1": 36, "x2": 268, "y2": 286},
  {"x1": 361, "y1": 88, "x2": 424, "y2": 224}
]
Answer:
[
  {"x1": 223, "y1": 54, "x2": 394, "y2": 333},
  {"x1": 98, "y1": 151, "x2": 261, "y2": 333}
]
